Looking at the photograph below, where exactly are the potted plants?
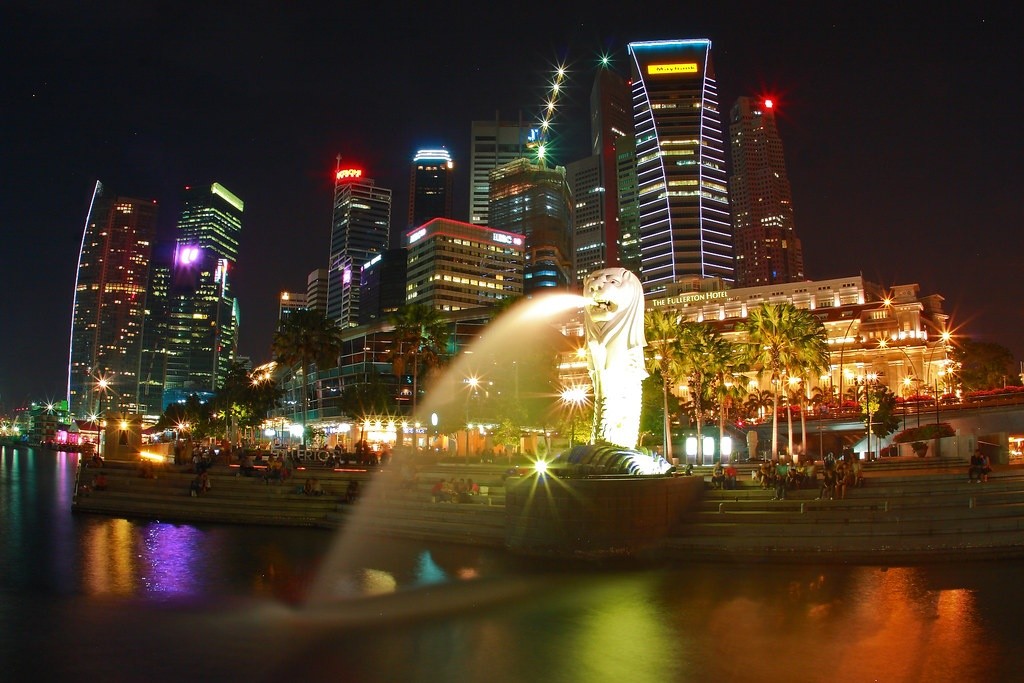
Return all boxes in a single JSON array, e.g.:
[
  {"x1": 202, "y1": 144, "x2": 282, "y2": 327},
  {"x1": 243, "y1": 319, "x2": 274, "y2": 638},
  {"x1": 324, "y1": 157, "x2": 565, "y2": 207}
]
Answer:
[{"x1": 912, "y1": 442, "x2": 928, "y2": 457}]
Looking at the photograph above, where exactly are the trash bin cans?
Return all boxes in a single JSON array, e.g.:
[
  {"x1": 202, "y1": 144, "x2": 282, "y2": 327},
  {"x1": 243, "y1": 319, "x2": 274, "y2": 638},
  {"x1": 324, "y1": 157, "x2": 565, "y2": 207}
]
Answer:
[
  {"x1": 889, "y1": 444, "x2": 898, "y2": 457},
  {"x1": 865, "y1": 452, "x2": 875, "y2": 462}
]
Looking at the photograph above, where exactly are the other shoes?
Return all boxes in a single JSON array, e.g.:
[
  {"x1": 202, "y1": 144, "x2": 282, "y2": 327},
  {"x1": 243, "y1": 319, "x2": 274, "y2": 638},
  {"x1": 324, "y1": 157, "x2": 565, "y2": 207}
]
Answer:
[
  {"x1": 780, "y1": 497, "x2": 788, "y2": 500},
  {"x1": 830, "y1": 497, "x2": 840, "y2": 500},
  {"x1": 967, "y1": 480, "x2": 972, "y2": 483},
  {"x1": 815, "y1": 497, "x2": 821, "y2": 500},
  {"x1": 771, "y1": 496, "x2": 782, "y2": 500},
  {"x1": 721, "y1": 487, "x2": 724, "y2": 490},
  {"x1": 713, "y1": 487, "x2": 717, "y2": 490},
  {"x1": 977, "y1": 480, "x2": 980, "y2": 484}
]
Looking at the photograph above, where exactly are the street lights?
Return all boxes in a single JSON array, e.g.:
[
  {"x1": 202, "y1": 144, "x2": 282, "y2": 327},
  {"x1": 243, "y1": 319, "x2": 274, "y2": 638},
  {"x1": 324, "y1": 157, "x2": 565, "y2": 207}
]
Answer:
[
  {"x1": 839, "y1": 298, "x2": 893, "y2": 409},
  {"x1": 927, "y1": 332, "x2": 952, "y2": 387},
  {"x1": 96, "y1": 379, "x2": 107, "y2": 457},
  {"x1": 878, "y1": 338, "x2": 919, "y2": 387}
]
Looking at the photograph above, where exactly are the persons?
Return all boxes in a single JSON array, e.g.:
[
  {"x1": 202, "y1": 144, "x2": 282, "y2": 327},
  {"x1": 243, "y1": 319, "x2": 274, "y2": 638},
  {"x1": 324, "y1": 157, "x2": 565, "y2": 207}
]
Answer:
[
  {"x1": 754, "y1": 447, "x2": 859, "y2": 500},
  {"x1": 725, "y1": 463, "x2": 736, "y2": 490},
  {"x1": 91, "y1": 471, "x2": 108, "y2": 491},
  {"x1": 968, "y1": 449, "x2": 992, "y2": 483},
  {"x1": 91, "y1": 452, "x2": 105, "y2": 468},
  {"x1": 138, "y1": 437, "x2": 509, "y2": 504},
  {"x1": 711, "y1": 462, "x2": 725, "y2": 490}
]
